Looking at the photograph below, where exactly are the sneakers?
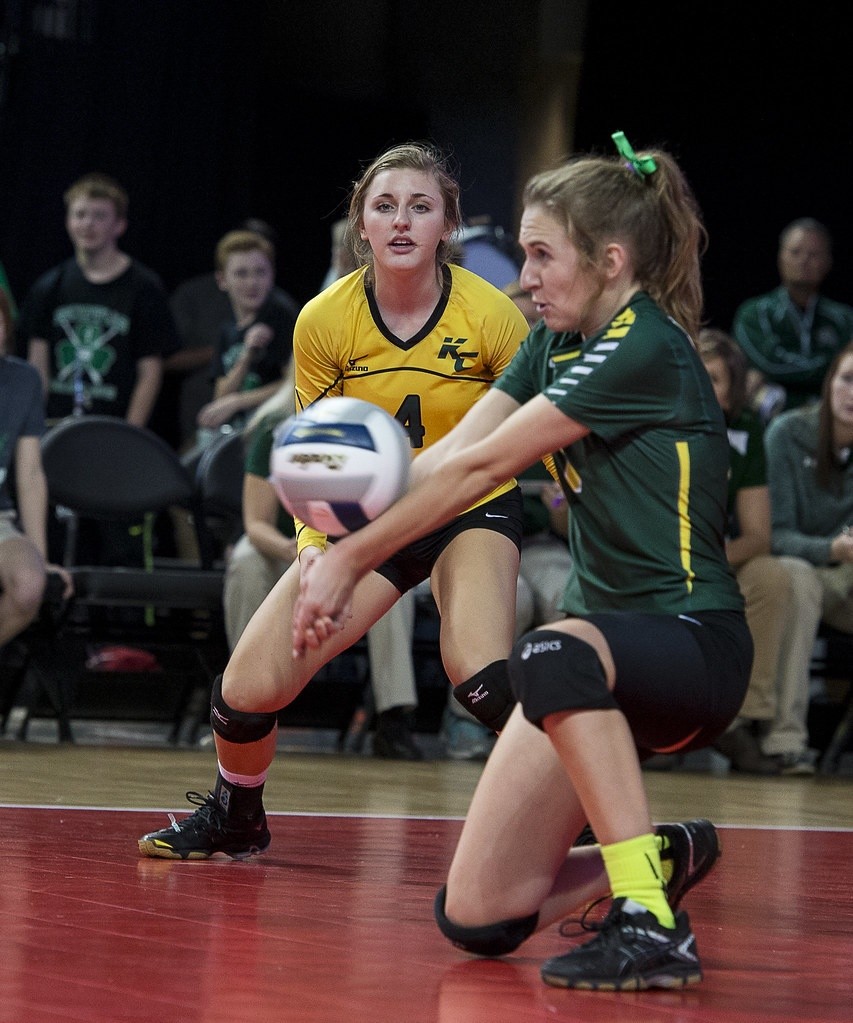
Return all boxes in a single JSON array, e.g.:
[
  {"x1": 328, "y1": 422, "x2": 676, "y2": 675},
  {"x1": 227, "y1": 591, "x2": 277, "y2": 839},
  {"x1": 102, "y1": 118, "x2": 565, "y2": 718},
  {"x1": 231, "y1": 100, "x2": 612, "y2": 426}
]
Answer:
[
  {"x1": 138, "y1": 773, "x2": 271, "y2": 861},
  {"x1": 655, "y1": 817, "x2": 722, "y2": 915},
  {"x1": 540, "y1": 893, "x2": 704, "y2": 991}
]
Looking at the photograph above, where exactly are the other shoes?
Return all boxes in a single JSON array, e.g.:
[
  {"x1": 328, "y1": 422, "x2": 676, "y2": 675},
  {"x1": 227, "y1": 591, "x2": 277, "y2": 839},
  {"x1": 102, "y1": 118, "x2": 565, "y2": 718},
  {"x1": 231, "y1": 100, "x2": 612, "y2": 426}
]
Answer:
[
  {"x1": 371, "y1": 721, "x2": 427, "y2": 762},
  {"x1": 436, "y1": 709, "x2": 496, "y2": 760},
  {"x1": 760, "y1": 749, "x2": 818, "y2": 776}
]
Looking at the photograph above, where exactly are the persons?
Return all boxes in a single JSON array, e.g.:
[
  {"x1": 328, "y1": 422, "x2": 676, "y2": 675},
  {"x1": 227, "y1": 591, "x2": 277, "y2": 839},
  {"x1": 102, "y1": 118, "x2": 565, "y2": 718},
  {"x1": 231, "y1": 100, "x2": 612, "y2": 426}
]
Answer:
[
  {"x1": 290, "y1": 130, "x2": 758, "y2": 990},
  {"x1": 0, "y1": 143, "x2": 852, "y2": 860}
]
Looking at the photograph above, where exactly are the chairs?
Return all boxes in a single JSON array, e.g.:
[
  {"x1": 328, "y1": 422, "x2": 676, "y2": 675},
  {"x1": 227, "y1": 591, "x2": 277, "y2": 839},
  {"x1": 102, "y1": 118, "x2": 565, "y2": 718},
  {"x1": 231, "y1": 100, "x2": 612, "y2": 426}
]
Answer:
[{"x1": 0, "y1": 420, "x2": 368, "y2": 755}]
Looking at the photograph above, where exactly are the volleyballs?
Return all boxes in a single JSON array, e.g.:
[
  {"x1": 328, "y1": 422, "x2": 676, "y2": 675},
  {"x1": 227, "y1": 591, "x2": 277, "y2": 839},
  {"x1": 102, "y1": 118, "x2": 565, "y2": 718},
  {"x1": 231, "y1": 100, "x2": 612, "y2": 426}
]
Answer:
[{"x1": 268, "y1": 395, "x2": 409, "y2": 538}]
